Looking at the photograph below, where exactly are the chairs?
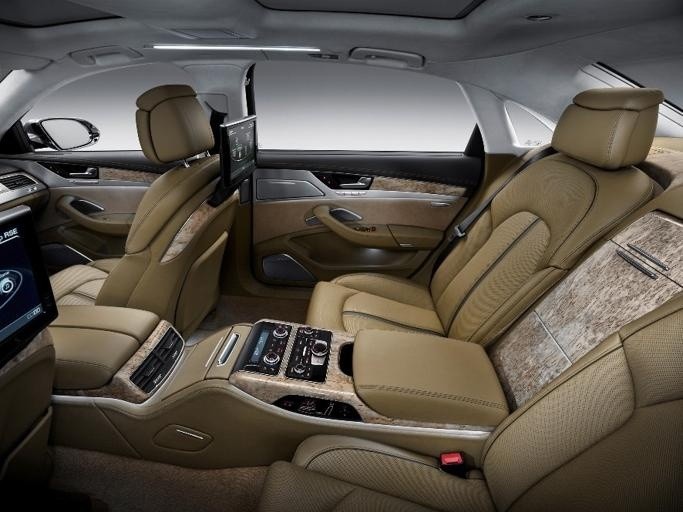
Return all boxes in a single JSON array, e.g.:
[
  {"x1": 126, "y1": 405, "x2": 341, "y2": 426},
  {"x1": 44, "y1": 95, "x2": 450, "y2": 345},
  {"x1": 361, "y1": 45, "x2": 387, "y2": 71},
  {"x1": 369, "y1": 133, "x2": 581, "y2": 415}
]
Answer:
[
  {"x1": 242, "y1": 291, "x2": 682, "y2": 512},
  {"x1": 42, "y1": 77, "x2": 239, "y2": 344},
  {"x1": 298, "y1": 85, "x2": 666, "y2": 351},
  {"x1": 0, "y1": 180, "x2": 62, "y2": 511}
]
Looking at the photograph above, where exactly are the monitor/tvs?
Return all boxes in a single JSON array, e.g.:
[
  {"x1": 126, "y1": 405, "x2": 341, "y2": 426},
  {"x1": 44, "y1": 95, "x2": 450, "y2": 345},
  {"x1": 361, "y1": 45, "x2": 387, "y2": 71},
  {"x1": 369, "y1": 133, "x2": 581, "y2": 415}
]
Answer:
[
  {"x1": 0, "y1": 205, "x2": 59, "y2": 370},
  {"x1": 219, "y1": 115, "x2": 258, "y2": 192}
]
